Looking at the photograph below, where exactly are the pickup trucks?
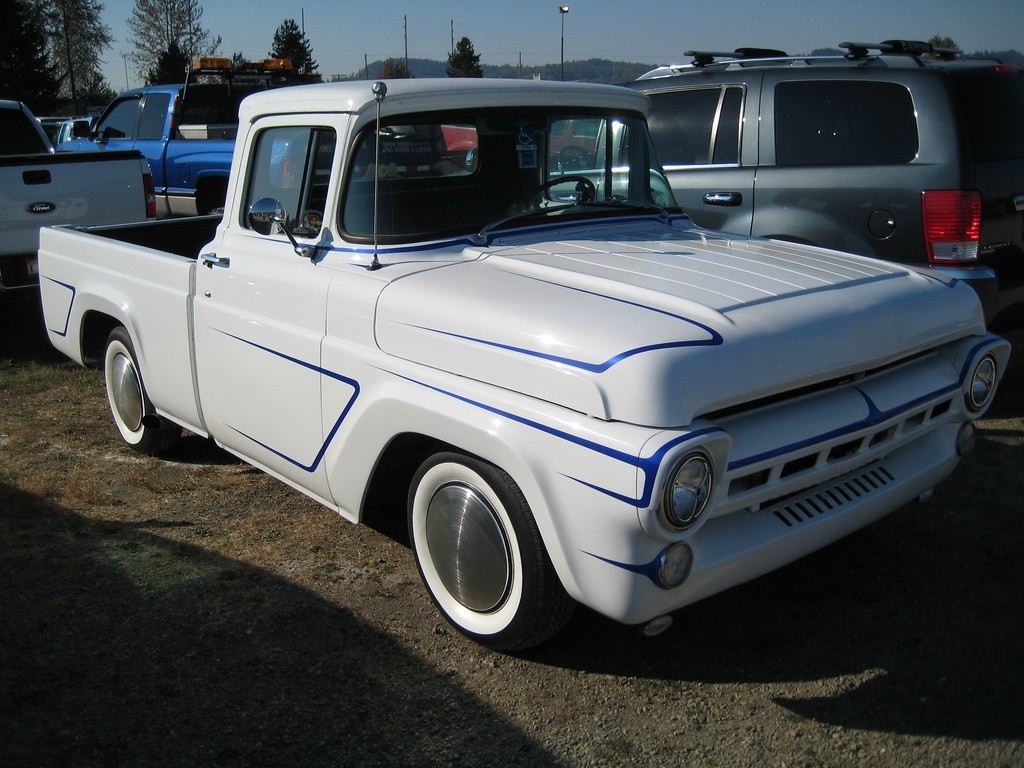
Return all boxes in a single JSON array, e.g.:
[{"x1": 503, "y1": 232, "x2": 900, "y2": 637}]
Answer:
[
  {"x1": 38, "y1": 78, "x2": 1013, "y2": 654},
  {"x1": 440, "y1": 116, "x2": 602, "y2": 168},
  {"x1": 0, "y1": 99, "x2": 157, "y2": 291},
  {"x1": 58, "y1": 86, "x2": 241, "y2": 215}
]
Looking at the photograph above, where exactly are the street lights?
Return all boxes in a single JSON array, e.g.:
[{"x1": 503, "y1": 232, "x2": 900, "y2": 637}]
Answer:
[{"x1": 559, "y1": 7, "x2": 569, "y2": 81}]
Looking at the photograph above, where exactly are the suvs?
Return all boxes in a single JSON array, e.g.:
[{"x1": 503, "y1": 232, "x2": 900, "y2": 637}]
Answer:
[{"x1": 550, "y1": 42, "x2": 1024, "y2": 324}]
